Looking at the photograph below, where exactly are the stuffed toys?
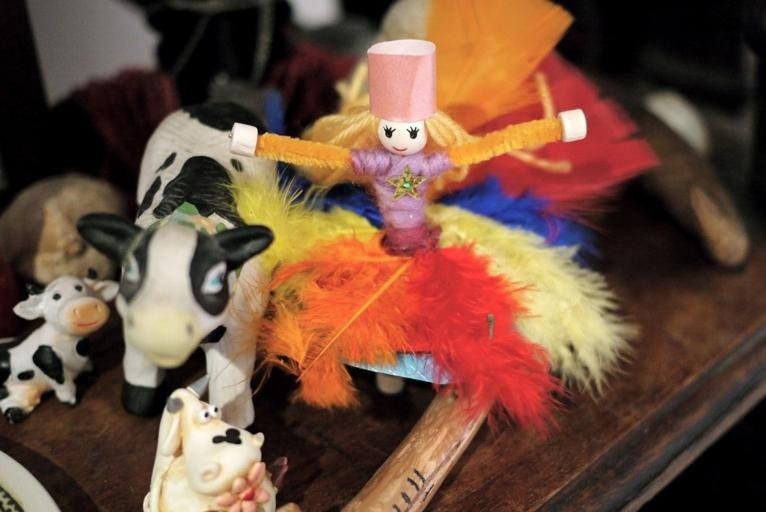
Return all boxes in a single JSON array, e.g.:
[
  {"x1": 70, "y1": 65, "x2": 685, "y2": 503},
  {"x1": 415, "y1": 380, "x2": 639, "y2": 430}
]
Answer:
[{"x1": 1, "y1": 176, "x2": 127, "y2": 320}]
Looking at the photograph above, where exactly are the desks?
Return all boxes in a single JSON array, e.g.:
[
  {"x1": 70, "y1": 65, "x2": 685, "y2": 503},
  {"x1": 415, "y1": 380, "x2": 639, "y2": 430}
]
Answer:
[{"x1": 0, "y1": 180, "x2": 766, "y2": 511}]
